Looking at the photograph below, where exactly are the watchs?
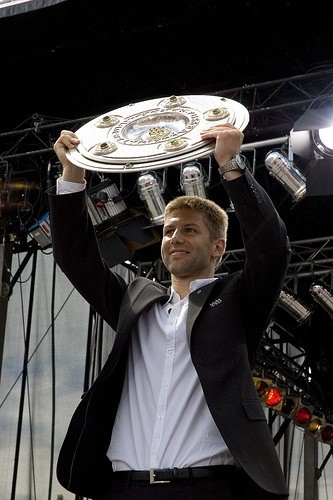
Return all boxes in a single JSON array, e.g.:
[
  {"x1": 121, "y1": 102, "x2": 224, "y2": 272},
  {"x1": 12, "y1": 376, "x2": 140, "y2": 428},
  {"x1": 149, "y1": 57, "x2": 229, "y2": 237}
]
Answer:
[{"x1": 217, "y1": 153, "x2": 247, "y2": 176}]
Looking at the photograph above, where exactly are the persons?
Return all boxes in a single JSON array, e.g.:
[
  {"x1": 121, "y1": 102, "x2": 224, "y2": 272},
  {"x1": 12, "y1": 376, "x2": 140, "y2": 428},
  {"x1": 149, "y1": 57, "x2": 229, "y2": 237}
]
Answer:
[{"x1": 48, "y1": 121, "x2": 292, "y2": 500}]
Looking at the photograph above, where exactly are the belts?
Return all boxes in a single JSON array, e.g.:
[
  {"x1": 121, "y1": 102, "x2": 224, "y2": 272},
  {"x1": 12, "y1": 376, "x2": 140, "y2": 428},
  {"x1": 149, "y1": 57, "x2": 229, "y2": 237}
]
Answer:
[{"x1": 111, "y1": 465, "x2": 246, "y2": 484}]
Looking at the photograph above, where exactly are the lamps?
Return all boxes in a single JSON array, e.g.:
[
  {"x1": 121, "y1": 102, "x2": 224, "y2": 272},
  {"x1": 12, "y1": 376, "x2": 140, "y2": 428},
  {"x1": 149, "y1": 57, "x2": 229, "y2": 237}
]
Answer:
[
  {"x1": 137, "y1": 166, "x2": 167, "y2": 226},
  {"x1": 179, "y1": 157, "x2": 212, "y2": 199},
  {"x1": 84, "y1": 177, "x2": 135, "y2": 240},
  {"x1": 228, "y1": 147, "x2": 256, "y2": 213},
  {"x1": 311, "y1": 128, "x2": 333, "y2": 160},
  {"x1": 309, "y1": 280, "x2": 333, "y2": 319},
  {"x1": 276, "y1": 290, "x2": 313, "y2": 324},
  {"x1": 252, "y1": 376, "x2": 333, "y2": 448},
  {"x1": 263, "y1": 138, "x2": 313, "y2": 204}
]
